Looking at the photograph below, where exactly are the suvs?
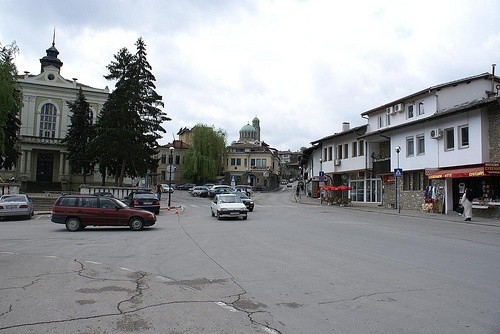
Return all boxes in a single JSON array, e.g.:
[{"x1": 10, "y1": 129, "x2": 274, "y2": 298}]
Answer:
[{"x1": 50, "y1": 193, "x2": 158, "y2": 231}]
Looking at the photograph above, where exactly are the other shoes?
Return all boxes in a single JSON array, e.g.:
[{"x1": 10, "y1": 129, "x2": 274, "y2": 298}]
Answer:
[{"x1": 464, "y1": 218, "x2": 471, "y2": 221}]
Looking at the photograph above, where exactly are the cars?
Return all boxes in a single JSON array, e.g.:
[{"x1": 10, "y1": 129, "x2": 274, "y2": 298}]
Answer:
[
  {"x1": 281, "y1": 177, "x2": 297, "y2": 188},
  {"x1": 157, "y1": 183, "x2": 174, "y2": 193},
  {"x1": 229, "y1": 191, "x2": 254, "y2": 212},
  {"x1": 176, "y1": 183, "x2": 196, "y2": 190},
  {"x1": 0, "y1": 193, "x2": 34, "y2": 221},
  {"x1": 189, "y1": 182, "x2": 253, "y2": 199},
  {"x1": 210, "y1": 193, "x2": 248, "y2": 221},
  {"x1": 94, "y1": 192, "x2": 115, "y2": 198},
  {"x1": 124, "y1": 191, "x2": 161, "y2": 215}
]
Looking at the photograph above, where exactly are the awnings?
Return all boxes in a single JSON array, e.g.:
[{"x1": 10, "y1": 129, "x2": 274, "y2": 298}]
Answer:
[{"x1": 428, "y1": 166, "x2": 489, "y2": 179}]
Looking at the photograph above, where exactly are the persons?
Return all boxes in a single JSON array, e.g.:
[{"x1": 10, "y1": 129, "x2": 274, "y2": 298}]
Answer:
[{"x1": 462, "y1": 186, "x2": 472, "y2": 221}]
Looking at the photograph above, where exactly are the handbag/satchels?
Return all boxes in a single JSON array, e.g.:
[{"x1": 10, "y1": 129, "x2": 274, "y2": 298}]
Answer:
[{"x1": 455, "y1": 204, "x2": 464, "y2": 214}]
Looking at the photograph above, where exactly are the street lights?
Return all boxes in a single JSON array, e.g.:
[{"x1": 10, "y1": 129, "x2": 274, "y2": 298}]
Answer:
[{"x1": 168, "y1": 147, "x2": 174, "y2": 207}]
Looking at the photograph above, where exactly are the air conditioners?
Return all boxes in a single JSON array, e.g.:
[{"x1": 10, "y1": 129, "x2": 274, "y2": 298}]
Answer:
[
  {"x1": 430, "y1": 128, "x2": 442, "y2": 140},
  {"x1": 394, "y1": 103, "x2": 404, "y2": 113},
  {"x1": 334, "y1": 159, "x2": 341, "y2": 166},
  {"x1": 386, "y1": 106, "x2": 395, "y2": 115}
]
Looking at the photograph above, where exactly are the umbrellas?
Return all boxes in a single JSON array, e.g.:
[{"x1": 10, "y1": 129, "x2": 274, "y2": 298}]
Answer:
[
  {"x1": 334, "y1": 185, "x2": 353, "y2": 199},
  {"x1": 320, "y1": 185, "x2": 335, "y2": 197}
]
